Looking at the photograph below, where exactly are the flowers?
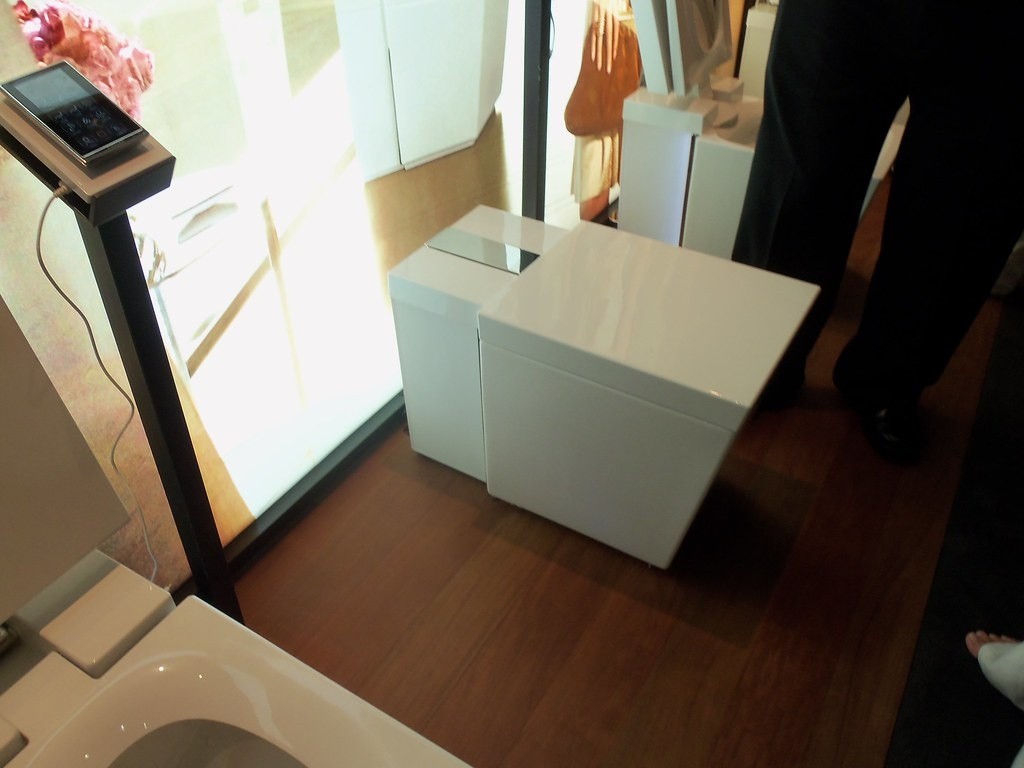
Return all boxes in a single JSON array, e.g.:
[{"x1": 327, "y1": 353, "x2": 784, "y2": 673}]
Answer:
[{"x1": 13, "y1": 0, "x2": 153, "y2": 123}]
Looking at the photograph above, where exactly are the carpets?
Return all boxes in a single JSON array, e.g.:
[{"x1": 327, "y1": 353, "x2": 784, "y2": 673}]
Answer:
[{"x1": 882, "y1": 291, "x2": 1024, "y2": 768}]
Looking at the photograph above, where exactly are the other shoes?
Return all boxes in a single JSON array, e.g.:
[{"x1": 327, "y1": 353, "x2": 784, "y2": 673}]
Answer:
[
  {"x1": 839, "y1": 369, "x2": 927, "y2": 467},
  {"x1": 756, "y1": 361, "x2": 802, "y2": 411}
]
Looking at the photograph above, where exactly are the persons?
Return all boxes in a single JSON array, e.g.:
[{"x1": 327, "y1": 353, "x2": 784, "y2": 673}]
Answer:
[{"x1": 731, "y1": 0, "x2": 1024, "y2": 470}]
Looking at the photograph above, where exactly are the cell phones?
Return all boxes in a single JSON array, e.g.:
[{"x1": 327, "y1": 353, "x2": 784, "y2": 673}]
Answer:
[{"x1": 0, "y1": 60, "x2": 149, "y2": 166}]
